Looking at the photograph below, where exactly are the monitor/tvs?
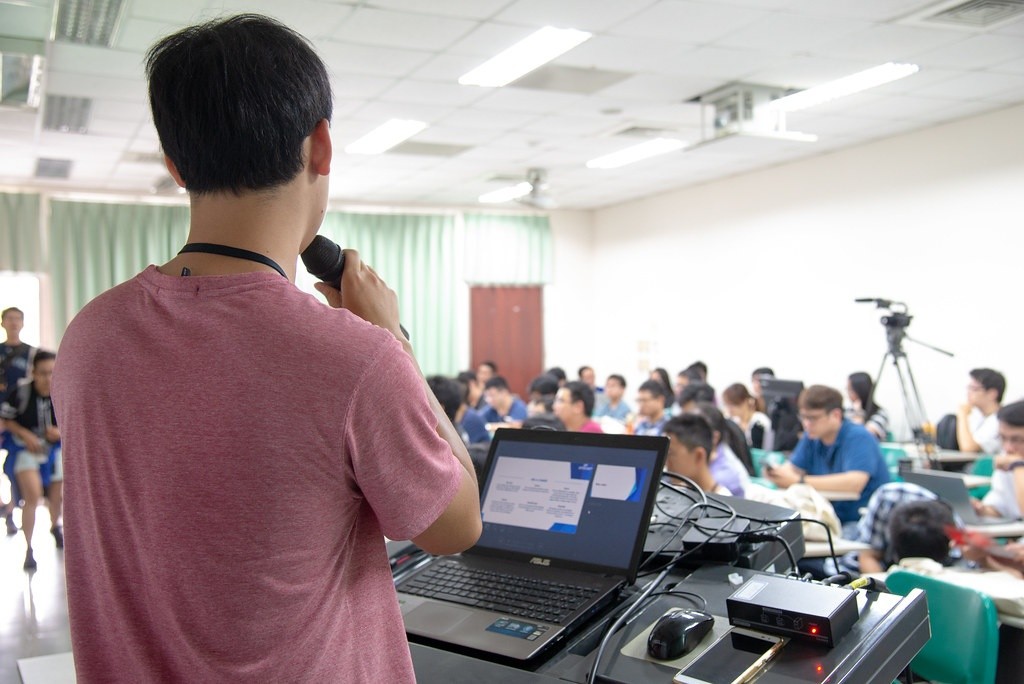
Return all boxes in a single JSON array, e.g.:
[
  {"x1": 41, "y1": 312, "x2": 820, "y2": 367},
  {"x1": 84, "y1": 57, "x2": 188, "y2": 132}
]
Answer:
[{"x1": 758, "y1": 379, "x2": 806, "y2": 431}]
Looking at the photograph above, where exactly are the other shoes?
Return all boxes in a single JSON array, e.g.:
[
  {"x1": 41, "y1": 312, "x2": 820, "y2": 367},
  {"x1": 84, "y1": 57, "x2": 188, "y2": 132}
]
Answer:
[
  {"x1": 24, "y1": 555, "x2": 36, "y2": 571},
  {"x1": 50, "y1": 525, "x2": 63, "y2": 547},
  {"x1": 6, "y1": 515, "x2": 17, "y2": 535}
]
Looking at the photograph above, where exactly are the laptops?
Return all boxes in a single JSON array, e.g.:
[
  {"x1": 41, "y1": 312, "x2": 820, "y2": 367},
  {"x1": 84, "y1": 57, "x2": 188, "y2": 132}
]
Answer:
[
  {"x1": 902, "y1": 469, "x2": 1014, "y2": 525},
  {"x1": 391, "y1": 426, "x2": 669, "y2": 671}
]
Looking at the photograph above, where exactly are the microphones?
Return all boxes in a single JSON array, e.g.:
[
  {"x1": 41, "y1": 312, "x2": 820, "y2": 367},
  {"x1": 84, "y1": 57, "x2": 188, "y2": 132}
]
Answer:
[{"x1": 301, "y1": 236, "x2": 408, "y2": 342}]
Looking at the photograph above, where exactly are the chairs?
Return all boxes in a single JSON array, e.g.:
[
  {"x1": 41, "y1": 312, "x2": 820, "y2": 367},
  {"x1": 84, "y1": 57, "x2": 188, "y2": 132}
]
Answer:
[
  {"x1": 964, "y1": 457, "x2": 1008, "y2": 545},
  {"x1": 885, "y1": 572, "x2": 999, "y2": 684},
  {"x1": 878, "y1": 443, "x2": 908, "y2": 482},
  {"x1": 746, "y1": 447, "x2": 785, "y2": 490}
]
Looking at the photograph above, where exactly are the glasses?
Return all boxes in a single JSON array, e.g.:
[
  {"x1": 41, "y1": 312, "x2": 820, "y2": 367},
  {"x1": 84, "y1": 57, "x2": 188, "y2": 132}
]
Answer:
[
  {"x1": 635, "y1": 397, "x2": 653, "y2": 404},
  {"x1": 796, "y1": 413, "x2": 831, "y2": 422},
  {"x1": 994, "y1": 433, "x2": 1024, "y2": 444},
  {"x1": 967, "y1": 385, "x2": 983, "y2": 391}
]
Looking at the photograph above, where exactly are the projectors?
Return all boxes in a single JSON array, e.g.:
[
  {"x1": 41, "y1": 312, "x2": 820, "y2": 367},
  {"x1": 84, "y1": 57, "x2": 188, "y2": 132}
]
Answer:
[{"x1": 716, "y1": 108, "x2": 766, "y2": 132}]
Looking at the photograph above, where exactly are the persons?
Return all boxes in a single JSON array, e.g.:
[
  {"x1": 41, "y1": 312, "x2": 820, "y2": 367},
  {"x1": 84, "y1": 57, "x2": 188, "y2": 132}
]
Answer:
[
  {"x1": 48, "y1": 12, "x2": 483, "y2": 684},
  {"x1": 0, "y1": 307, "x2": 64, "y2": 569},
  {"x1": 418, "y1": 358, "x2": 1024, "y2": 574}
]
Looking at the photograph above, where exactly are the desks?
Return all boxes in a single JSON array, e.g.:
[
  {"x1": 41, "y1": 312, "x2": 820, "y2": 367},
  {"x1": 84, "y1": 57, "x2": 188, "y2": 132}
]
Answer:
[
  {"x1": 900, "y1": 440, "x2": 980, "y2": 469},
  {"x1": 922, "y1": 471, "x2": 992, "y2": 488},
  {"x1": 797, "y1": 559, "x2": 1024, "y2": 630}
]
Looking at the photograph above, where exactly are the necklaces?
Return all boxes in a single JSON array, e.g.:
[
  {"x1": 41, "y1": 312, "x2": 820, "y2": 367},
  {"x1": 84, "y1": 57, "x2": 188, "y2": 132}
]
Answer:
[
  {"x1": 1008, "y1": 461, "x2": 1024, "y2": 470},
  {"x1": 175, "y1": 243, "x2": 290, "y2": 280}
]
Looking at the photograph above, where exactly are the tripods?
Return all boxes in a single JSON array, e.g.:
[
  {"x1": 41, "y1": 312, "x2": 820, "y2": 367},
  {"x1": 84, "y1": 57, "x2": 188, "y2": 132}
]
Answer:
[{"x1": 864, "y1": 328, "x2": 953, "y2": 470}]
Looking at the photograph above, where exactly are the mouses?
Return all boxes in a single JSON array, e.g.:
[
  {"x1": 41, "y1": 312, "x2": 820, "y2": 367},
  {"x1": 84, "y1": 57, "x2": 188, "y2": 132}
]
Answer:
[{"x1": 647, "y1": 607, "x2": 714, "y2": 661}]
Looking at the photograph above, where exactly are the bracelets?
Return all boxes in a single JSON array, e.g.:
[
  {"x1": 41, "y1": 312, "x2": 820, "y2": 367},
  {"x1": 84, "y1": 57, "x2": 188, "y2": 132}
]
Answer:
[{"x1": 800, "y1": 474, "x2": 805, "y2": 483}]
